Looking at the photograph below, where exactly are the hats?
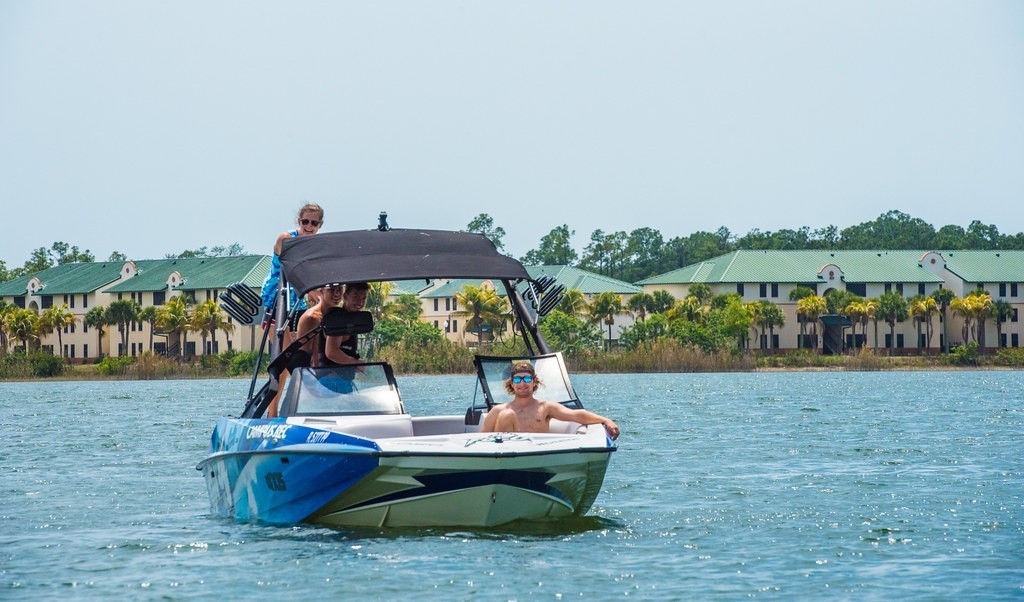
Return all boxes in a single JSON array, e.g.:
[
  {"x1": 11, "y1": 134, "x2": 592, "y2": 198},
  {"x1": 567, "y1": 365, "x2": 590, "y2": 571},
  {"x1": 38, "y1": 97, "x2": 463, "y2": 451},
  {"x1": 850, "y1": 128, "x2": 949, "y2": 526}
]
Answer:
[{"x1": 511, "y1": 362, "x2": 536, "y2": 375}]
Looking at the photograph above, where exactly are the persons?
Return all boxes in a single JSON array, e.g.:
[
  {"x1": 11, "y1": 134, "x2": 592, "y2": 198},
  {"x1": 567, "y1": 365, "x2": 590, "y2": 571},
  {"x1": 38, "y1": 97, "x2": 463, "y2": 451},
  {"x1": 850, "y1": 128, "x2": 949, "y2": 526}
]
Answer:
[
  {"x1": 296, "y1": 280, "x2": 371, "y2": 399},
  {"x1": 261, "y1": 203, "x2": 325, "y2": 418},
  {"x1": 479, "y1": 362, "x2": 621, "y2": 441}
]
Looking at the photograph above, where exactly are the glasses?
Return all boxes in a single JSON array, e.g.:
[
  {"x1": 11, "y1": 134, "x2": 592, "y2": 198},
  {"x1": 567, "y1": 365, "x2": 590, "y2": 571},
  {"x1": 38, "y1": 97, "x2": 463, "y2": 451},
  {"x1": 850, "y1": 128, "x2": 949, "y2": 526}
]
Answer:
[
  {"x1": 512, "y1": 375, "x2": 536, "y2": 383},
  {"x1": 298, "y1": 219, "x2": 322, "y2": 226}
]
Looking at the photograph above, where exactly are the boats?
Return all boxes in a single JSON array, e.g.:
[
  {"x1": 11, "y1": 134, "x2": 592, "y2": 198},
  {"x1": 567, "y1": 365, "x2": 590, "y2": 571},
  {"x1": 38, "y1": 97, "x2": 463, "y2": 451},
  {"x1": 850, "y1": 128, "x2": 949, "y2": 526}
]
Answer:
[{"x1": 196, "y1": 213, "x2": 620, "y2": 532}]
